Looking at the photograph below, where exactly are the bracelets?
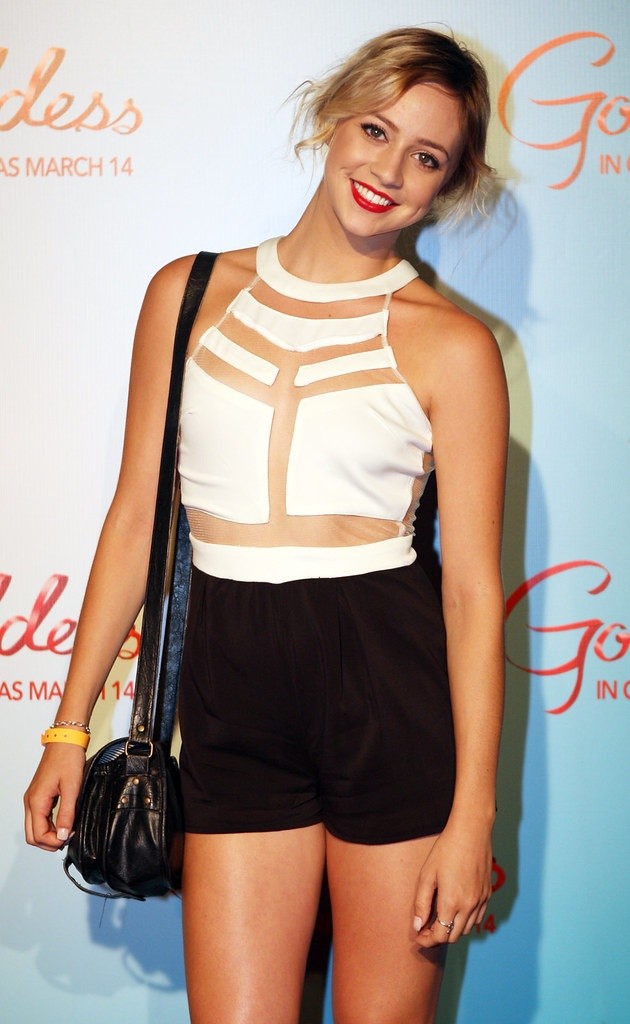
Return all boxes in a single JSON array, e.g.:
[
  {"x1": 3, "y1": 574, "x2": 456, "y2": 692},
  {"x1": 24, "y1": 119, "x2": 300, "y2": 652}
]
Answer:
[
  {"x1": 41, "y1": 729, "x2": 90, "y2": 752},
  {"x1": 50, "y1": 721, "x2": 91, "y2": 733}
]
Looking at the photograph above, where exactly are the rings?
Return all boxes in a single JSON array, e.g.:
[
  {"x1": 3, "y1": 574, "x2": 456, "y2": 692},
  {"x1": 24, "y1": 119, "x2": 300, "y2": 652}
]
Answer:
[{"x1": 437, "y1": 919, "x2": 454, "y2": 934}]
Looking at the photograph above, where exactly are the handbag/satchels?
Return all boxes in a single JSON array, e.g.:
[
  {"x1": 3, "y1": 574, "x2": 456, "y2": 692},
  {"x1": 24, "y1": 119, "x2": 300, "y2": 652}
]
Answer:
[{"x1": 64, "y1": 738, "x2": 183, "y2": 903}]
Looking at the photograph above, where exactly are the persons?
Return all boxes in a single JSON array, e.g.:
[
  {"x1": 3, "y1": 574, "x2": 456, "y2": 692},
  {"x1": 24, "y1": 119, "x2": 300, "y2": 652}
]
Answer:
[{"x1": 22, "y1": 23, "x2": 512, "y2": 1024}]
